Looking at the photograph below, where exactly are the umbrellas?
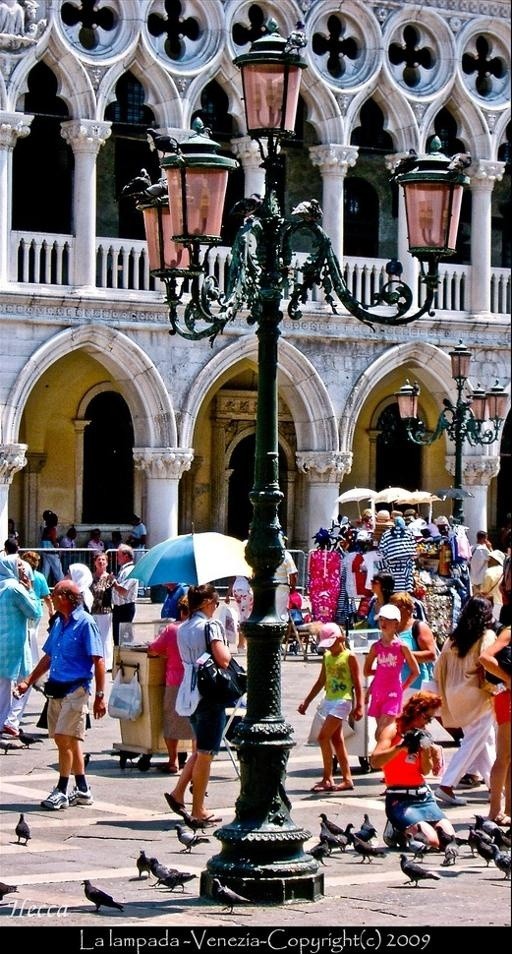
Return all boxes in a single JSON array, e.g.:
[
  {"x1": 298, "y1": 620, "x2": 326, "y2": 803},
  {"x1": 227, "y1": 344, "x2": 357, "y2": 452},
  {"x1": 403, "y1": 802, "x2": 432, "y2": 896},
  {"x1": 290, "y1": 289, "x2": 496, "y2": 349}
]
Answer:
[
  {"x1": 368, "y1": 485, "x2": 417, "y2": 512},
  {"x1": 431, "y1": 484, "x2": 477, "y2": 515},
  {"x1": 334, "y1": 486, "x2": 378, "y2": 521},
  {"x1": 395, "y1": 489, "x2": 443, "y2": 517}
]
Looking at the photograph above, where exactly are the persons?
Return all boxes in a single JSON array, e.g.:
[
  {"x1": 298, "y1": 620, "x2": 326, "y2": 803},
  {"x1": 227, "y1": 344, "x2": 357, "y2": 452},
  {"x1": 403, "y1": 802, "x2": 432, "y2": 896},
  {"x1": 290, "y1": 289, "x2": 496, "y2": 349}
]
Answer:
[{"x1": 2, "y1": 511, "x2": 512, "y2": 853}]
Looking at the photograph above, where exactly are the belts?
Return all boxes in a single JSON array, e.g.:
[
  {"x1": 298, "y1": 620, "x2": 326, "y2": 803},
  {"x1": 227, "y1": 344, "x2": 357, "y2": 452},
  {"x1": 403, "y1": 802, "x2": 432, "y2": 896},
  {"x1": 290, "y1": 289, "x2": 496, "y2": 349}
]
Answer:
[{"x1": 386, "y1": 786, "x2": 427, "y2": 795}]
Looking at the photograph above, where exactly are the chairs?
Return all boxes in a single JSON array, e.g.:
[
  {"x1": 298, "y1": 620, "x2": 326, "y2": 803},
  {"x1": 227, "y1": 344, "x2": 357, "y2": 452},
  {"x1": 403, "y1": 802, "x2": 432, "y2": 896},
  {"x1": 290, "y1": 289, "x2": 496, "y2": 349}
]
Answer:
[
  {"x1": 219, "y1": 693, "x2": 247, "y2": 779},
  {"x1": 283, "y1": 606, "x2": 322, "y2": 660}
]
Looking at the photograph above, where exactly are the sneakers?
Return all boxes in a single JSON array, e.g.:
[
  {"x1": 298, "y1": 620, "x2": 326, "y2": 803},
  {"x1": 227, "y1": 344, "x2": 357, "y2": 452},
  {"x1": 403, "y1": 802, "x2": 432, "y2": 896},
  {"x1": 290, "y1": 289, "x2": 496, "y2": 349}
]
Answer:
[
  {"x1": 67, "y1": 784, "x2": 93, "y2": 806},
  {"x1": 383, "y1": 820, "x2": 398, "y2": 848},
  {"x1": 40, "y1": 788, "x2": 69, "y2": 810}
]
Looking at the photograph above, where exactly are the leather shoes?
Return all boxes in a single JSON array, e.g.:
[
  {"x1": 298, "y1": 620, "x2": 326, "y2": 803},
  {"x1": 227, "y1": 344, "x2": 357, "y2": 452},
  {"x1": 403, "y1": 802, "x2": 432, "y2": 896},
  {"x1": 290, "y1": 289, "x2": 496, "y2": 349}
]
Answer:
[{"x1": 435, "y1": 787, "x2": 466, "y2": 805}]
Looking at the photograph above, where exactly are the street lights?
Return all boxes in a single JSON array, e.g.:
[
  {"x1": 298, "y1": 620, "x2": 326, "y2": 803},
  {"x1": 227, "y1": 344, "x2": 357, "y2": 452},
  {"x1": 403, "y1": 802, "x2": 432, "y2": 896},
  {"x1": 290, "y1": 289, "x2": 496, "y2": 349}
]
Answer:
[
  {"x1": 120, "y1": 17, "x2": 471, "y2": 908},
  {"x1": 392, "y1": 340, "x2": 508, "y2": 524}
]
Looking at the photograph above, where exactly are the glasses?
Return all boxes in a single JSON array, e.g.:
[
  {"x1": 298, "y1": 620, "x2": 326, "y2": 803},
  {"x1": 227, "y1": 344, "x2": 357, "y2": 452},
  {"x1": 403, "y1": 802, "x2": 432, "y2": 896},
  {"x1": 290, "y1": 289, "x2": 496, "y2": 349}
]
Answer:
[{"x1": 215, "y1": 600, "x2": 220, "y2": 609}]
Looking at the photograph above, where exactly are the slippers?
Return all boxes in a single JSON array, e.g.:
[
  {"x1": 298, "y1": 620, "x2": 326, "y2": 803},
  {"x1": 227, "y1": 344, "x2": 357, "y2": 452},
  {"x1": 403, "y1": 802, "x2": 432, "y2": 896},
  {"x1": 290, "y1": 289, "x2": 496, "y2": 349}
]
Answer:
[
  {"x1": 309, "y1": 776, "x2": 333, "y2": 793},
  {"x1": 459, "y1": 777, "x2": 481, "y2": 787},
  {"x1": 491, "y1": 813, "x2": 511, "y2": 827},
  {"x1": 164, "y1": 792, "x2": 188, "y2": 817},
  {"x1": 331, "y1": 785, "x2": 354, "y2": 791},
  {"x1": 1, "y1": 733, "x2": 18, "y2": 740},
  {"x1": 204, "y1": 814, "x2": 223, "y2": 823}
]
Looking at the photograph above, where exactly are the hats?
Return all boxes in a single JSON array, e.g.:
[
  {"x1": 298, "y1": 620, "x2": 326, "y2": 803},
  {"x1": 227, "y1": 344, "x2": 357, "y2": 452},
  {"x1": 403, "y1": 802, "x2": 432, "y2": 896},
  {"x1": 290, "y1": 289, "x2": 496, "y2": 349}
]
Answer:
[
  {"x1": 487, "y1": 549, "x2": 505, "y2": 566},
  {"x1": 373, "y1": 603, "x2": 402, "y2": 623},
  {"x1": 318, "y1": 622, "x2": 342, "y2": 648},
  {"x1": 361, "y1": 508, "x2": 416, "y2": 523}
]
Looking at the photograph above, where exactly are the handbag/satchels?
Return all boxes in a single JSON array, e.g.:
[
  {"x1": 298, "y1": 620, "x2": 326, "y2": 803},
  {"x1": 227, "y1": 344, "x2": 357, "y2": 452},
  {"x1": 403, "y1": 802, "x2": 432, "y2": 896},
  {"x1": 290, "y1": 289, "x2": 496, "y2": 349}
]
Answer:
[
  {"x1": 44, "y1": 678, "x2": 71, "y2": 698},
  {"x1": 412, "y1": 620, "x2": 441, "y2": 680},
  {"x1": 101, "y1": 580, "x2": 114, "y2": 607},
  {"x1": 196, "y1": 618, "x2": 246, "y2": 709},
  {"x1": 485, "y1": 646, "x2": 512, "y2": 685}
]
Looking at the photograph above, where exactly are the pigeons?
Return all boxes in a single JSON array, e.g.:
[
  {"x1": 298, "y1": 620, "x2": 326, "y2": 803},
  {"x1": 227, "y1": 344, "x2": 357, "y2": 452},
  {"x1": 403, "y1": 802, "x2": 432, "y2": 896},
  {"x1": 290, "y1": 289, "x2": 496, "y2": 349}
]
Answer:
[
  {"x1": 398, "y1": 813, "x2": 512, "y2": 887},
  {"x1": 15, "y1": 813, "x2": 31, "y2": 845},
  {"x1": 173, "y1": 810, "x2": 222, "y2": 854},
  {"x1": 306, "y1": 813, "x2": 387, "y2": 865},
  {"x1": 80, "y1": 879, "x2": 124, "y2": 913},
  {"x1": 0, "y1": 732, "x2": 18, "y2": 755},
  {"x1": 83, "y1": 754, "x2": 90, "y2": 770},
  {"x1": 212, "y1": 877, "x2": 255, "y2": 915},
  {"x1": 0, "y1": 881, "x2": 19, "y2": 902},
  {"x1": 136, "y1": 849, "x2": 198, "y2": 893},
  {"x1": 18, "y1": 729, "x2": 44, "y2": 748}
]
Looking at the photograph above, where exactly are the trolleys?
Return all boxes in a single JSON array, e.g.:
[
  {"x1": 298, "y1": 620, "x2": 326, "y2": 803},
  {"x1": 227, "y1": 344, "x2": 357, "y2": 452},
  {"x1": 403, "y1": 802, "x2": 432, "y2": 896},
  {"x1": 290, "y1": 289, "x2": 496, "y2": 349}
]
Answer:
[{"x1": 113, "y1": 619, "x2": 197, "y2": 771}]
